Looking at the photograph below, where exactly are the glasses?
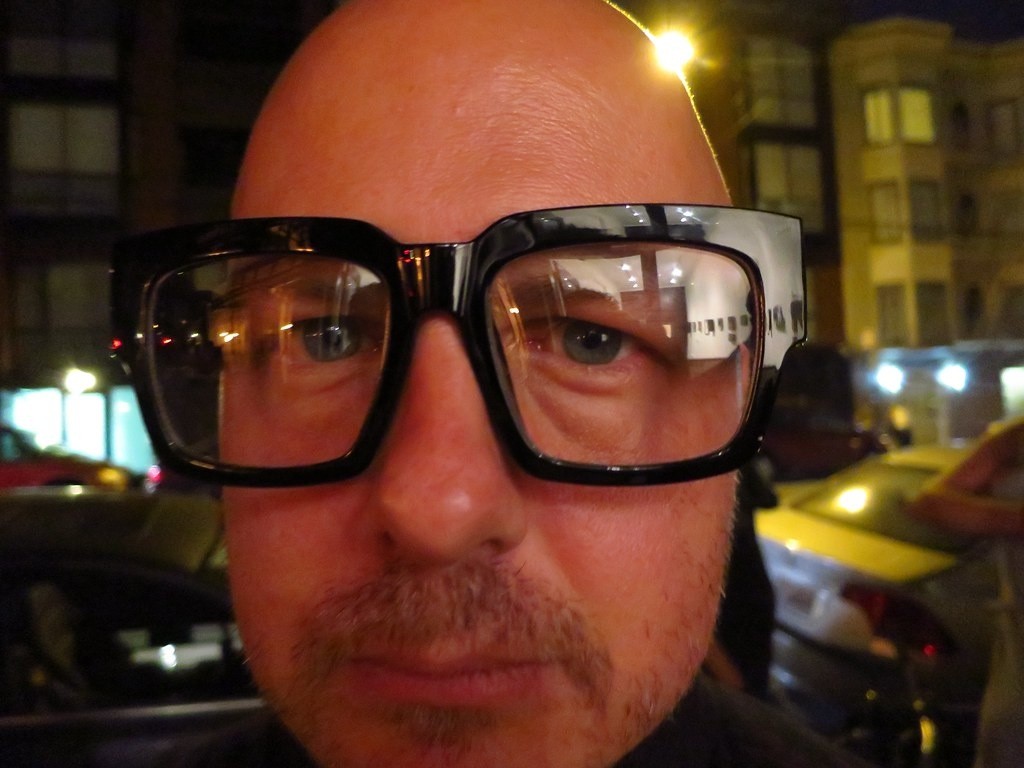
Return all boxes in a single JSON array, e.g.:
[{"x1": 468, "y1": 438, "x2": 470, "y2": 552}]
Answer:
[{"x1": 112, "y1": 201, "x2": 809, "y2": 489}]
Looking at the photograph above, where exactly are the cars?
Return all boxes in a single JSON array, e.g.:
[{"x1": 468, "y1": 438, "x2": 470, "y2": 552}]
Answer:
[
  {"x1": 751, "y1": 439, "x2": 1024, "y2": 766},
  {"x1": 0, "y1": 419, "x2": 147, "y2": 496}
]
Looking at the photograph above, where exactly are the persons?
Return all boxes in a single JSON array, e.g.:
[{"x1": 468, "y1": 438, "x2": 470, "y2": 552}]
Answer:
[
  {"x1": 905, "y1": 397, "x2": 1024, "y2": 768},
  {"x1": 108, "y1": 1, "x2": 887, "y2": 768}
]
